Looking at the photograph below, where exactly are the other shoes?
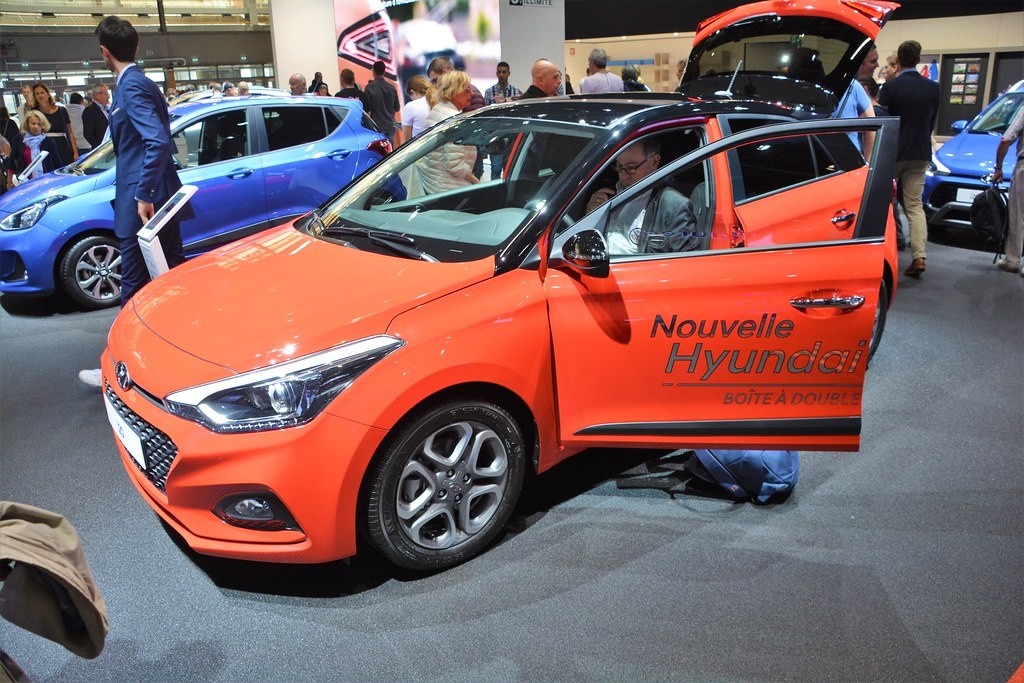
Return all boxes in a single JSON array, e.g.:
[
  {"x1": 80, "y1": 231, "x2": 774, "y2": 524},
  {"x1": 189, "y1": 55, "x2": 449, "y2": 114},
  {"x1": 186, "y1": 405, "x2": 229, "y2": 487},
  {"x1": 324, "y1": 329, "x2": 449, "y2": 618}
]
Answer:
[
  {"x1": 78, "y1": 368, "x2": 102, "y2": 388},
  {"x1": 997, "y1": 258, "x2": 1019, "y2": 273}
]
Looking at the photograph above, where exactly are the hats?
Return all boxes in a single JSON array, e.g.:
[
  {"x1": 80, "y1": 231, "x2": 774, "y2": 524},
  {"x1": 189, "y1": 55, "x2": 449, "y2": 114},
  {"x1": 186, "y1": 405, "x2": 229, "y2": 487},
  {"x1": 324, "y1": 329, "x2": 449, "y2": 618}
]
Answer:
[
  {"x1": 222, "y1": 83, "x2": 234, "y2": 92},
  {"x1": 0, "y1": 501, "x2": 110, "y2": 659},
  {"x1": 213, "y1": 83, "x2": 223, "y2": 92}
]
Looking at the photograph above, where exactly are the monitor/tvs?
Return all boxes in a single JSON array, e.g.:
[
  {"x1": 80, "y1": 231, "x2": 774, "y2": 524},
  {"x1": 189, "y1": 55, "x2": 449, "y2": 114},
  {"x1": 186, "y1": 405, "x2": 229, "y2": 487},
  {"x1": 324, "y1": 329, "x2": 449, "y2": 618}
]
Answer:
[
  {"x1": 22, "y1": 155, "x2": 42, "y2": 176},
  {"x1": 145, "y1": 193, "x2": 186, "y2": 230}
]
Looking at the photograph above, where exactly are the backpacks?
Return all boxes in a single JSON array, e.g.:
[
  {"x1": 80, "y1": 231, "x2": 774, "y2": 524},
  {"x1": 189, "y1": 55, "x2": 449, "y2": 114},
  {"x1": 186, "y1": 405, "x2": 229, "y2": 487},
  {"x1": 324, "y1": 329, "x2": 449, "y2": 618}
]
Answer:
[
  {"x1": 682, "y1": 437, "x2": 800, "y2": 504},
  {"x1": 970, "y1": 179, "x2": 1009, "y2": 264}
]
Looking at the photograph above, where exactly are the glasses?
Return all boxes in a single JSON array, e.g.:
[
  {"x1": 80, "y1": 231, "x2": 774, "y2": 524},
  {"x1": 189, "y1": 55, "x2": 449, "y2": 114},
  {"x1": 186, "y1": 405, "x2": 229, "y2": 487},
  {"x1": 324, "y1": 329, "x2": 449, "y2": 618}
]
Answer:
[{"x1": 610, "y1": 152, "x2": 661, "y2": 175}]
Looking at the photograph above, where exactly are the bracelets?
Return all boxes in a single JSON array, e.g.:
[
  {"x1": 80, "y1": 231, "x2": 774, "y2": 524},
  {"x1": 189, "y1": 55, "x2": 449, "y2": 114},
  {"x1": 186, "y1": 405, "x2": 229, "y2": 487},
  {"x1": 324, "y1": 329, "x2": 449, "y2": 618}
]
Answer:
[{"x1": 994, "y1": 166, "x2": 1003, "y2": 170}]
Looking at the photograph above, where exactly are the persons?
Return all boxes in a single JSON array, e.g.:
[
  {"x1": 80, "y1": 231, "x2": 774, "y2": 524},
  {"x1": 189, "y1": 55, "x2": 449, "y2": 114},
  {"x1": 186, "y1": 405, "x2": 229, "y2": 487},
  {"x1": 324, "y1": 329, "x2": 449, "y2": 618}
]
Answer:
[
  {"x1": 417, "y1": 70, "x2": 481, "y2": 195},
  {"x1": 79, "y1": 16, "x2": 197, "y2": 386},
  {"x1": 879, "y1": 41, "x2": 940, "y2": 277},
  {"x1": 992, "y1": 105, "x2": 1024, "y2": 276},
  {"x1": 402, "y1": 56, "x2": 564, "y2": 200},
  {"x1": 791, "y1": 40, "x2": 919, "y2": 250},
  {"x1": 289, "y1": 61, "x2": 400, "y2": 151},
  {"x1": 565, "y1": 48, "x2": 706, "y2": 94},
  {"x1": 0, "y1": 80, "x2": 249, "y2": 199},
  {"x1": 586, "y1": 140, "x2": 699, "y2": 258},
  {"x1": 1002, "y1": 83, "x2": 1016, "y2": 121}
]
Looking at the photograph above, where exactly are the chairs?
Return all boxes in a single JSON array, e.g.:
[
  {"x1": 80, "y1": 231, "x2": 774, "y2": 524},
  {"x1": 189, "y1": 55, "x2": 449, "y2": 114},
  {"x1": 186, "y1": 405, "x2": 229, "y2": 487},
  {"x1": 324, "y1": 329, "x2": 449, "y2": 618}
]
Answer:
[
  {"x1": 514, "y1": 136, "x2": 586, "y2": 207},
  {"x1": 216, "y1": 118, "x2": 247, "y2": 161},
  {"x1": 662, "y1": 132, "x2": 705, "y2": 197}
]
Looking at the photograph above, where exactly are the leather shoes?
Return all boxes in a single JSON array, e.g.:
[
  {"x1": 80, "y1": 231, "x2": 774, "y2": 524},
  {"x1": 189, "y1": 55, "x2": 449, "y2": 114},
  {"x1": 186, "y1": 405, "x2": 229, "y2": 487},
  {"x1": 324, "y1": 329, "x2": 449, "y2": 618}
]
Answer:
[{"x1": 904, "y1": 257, "x2": 926, "y2": 277}]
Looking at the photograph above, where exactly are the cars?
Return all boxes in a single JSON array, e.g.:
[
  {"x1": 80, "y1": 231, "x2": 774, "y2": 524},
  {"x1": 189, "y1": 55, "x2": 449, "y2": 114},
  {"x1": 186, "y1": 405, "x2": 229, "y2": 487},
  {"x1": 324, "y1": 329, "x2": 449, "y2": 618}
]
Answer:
[
  {"x1": 97, "y1": 0, "x2": 901, "y2": 578},
  {"x1": 0, "y1": 85, "x2": 408, "y2": 323},
  {"x1": 921, "y1": 80, "x2": 1023, "y2": 236}
]
